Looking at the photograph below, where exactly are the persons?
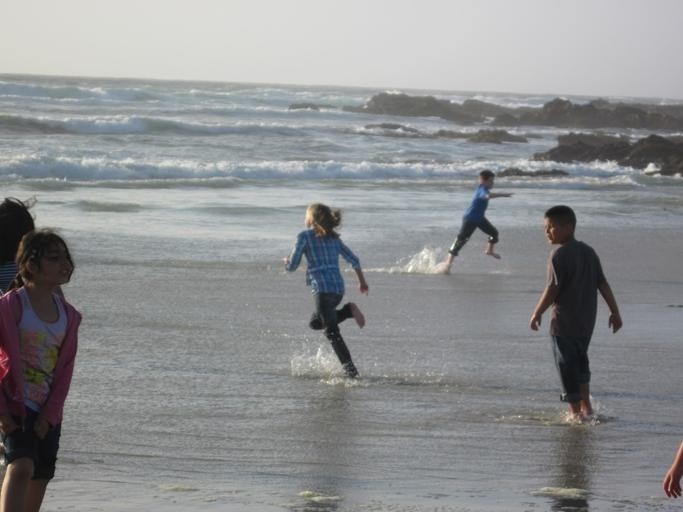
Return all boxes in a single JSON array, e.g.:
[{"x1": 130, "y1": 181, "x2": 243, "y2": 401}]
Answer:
[
  {"x1": 663, "y1": 442, "x2": 683, "y2": 499},
  {"x1": 531, "y1": 206, "x2": 622, "y2": 424},
  {"x1": 444, "y1": 170, "x2": 516, "y2": 276},
  {"x1": 282, "y1": 203, "x2": 369, "y2": 381},
  {"x1": 0, "y1": 197, "x2": 82, "y2": 512}
]
